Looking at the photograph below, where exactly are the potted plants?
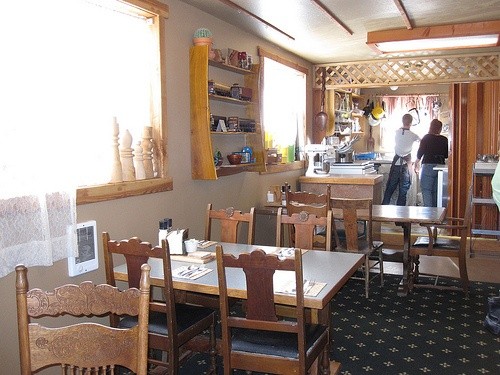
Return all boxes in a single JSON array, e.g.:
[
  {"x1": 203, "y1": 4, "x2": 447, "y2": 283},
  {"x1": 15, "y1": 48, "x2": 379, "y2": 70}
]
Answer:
[{"x1": 193, "y1": 28, "x2": 213, "y2": 57}]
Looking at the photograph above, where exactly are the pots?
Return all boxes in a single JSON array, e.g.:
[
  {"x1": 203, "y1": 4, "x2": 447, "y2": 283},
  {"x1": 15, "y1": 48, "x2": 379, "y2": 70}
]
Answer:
[{"x1": 336, "y1": 152, "x2": 353, "y2": 163}]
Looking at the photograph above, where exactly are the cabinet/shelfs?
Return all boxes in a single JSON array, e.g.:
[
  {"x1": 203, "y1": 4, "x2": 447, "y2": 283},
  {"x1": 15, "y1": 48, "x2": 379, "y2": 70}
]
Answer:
[
  {"x1": 326, "y1": 89, "x2": 365, "y2": 136},
  {"x1": 470, "y1": 163, "x2": 500, "y2": 258},
  {"x1": 299, "y1": 174, "x2": 383, "y2": 241},
  {"x1": 190, "y1": 44, "x2": 267, "y2": 180}
]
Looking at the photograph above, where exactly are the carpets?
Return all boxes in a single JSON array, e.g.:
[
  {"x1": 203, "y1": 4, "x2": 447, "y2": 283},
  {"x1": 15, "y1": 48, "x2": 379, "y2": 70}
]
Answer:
[{"x1": 120, "y1": 272, "x2": 500, "y2": 374}]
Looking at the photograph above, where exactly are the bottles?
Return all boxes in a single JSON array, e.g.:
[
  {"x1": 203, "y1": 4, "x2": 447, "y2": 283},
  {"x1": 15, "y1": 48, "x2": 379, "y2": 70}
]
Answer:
[
  {"x1": 164, "y1": 218, "x2": 172, "y2": 232},
  {"x1": 280, "y1": 185, "x2": 292, "y2": 207},
  {"x1": 231, "y1": 50, "x2": 253, "y2": 71},
  {"x1": 209, "y1": 79, "x2": 215, "y2": 94},
  {"x1": 230, "y1": 83, "x2": 241, "y2": 99},
  {"x1": 242, "y1": 146, "x2": 252, "y2": 162},
  {"x1": 158, "y1": 221, "x2": 168, "y2": 247},
  {"x1": 264, "y1": 132, "x2": 295, "y2": 164}
]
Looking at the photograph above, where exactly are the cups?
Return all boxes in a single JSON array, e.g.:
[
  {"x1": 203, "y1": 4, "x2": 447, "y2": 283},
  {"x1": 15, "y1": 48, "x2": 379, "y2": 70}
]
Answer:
[
  {"x1": 315, "y1": 161, "x2": 330, "y2": 173},
  {"x1": 226, "y1": 154, "x2": 243, "y2": 165}
]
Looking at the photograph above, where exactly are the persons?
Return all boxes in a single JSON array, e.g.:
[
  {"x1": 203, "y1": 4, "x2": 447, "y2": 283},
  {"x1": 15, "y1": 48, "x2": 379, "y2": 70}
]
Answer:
[
  {"x1": 381, "y1": 114, "x2": 421, "y2": 206},
  {"x1": 413, "y1": 119, "x2": 448, "y2": 208}
]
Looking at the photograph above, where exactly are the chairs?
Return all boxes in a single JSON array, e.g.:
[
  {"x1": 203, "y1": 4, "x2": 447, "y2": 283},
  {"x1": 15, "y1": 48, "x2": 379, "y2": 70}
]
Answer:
[
  {"x1": 15, "y1": 182, "x2": 383, "y2": 375},
  {"x1": 409, "y1": 186, "x2": 472, "y2": 297}
]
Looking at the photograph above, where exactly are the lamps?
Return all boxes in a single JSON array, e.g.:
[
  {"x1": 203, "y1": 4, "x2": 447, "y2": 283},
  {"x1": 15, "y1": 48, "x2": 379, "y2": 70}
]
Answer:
[{"x1": 364, "y1": 19, "x2": 500, "y2": 54}]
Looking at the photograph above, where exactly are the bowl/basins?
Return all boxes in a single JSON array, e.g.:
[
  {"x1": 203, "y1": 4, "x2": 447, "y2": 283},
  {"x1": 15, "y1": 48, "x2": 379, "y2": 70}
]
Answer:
[{"x1": 233, "y1": 151, "x2": 250, "y2": 164}]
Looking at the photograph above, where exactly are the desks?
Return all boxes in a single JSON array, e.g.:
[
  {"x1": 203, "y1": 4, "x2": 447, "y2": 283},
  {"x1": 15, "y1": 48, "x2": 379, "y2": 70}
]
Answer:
[
  {"x1": 115, "y1": 241, "x2": 366, "y2": 375},
  {"x1": 255, "y1": 205, "x2": 447, "y2": 297},
  {"x1": 433, "y1": 164, "x2": 449, "y2": 206}
]
